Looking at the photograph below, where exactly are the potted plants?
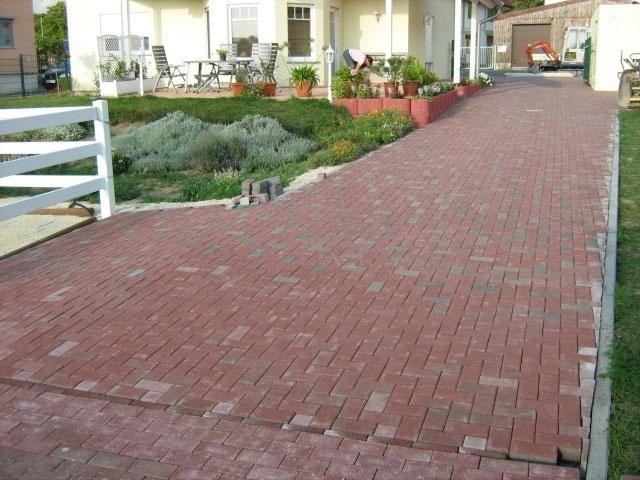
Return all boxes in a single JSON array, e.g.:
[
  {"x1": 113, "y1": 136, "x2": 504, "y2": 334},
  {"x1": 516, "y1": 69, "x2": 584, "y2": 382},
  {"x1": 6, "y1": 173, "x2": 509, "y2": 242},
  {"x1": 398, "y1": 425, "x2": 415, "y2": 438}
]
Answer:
[
  {"x1": 377, "y1": 56, "x2": 409, "y2": 98},
  {"x1": 287, "y1": 63, "x2": 320, "y2": 97},
  {"x1": 258, "y1": 57, "x2": 276, "y2": 96},
  {"x1": 402, "y1": 56, "x2": 438, "y2": 96},
  {"x1": 231, "y1": 66, "x2": 248, "y2": 96}
]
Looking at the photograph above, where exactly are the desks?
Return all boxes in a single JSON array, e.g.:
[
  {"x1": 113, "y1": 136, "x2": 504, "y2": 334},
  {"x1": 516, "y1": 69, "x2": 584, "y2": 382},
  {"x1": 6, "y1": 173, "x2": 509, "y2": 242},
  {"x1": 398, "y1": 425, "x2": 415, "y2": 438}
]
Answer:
[{"x1": 183, "y1": 58, "x2": 254, "y2": 94}]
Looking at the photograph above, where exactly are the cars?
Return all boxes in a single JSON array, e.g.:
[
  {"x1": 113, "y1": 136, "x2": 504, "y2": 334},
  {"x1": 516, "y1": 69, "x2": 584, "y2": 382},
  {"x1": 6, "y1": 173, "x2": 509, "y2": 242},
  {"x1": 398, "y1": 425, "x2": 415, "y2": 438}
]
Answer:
[{"x1": 41, "y1": 57, "x2": 70, "y2": 91}]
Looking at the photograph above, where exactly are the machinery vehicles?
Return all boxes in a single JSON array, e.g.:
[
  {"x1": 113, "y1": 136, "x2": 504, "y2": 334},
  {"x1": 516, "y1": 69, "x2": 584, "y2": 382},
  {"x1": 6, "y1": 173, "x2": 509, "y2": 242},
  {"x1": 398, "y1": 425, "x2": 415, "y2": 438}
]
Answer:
[{"x1": 524, "y1": 24, "x2": 590, "y2": 73}]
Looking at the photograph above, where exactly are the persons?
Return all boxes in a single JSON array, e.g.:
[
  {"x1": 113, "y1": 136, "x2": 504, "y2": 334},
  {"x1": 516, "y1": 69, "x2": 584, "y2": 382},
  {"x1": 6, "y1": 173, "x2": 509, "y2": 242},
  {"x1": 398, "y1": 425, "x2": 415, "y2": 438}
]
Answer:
[{"x1": 342, "y1": 47, "x2": 374, "y2": 70}]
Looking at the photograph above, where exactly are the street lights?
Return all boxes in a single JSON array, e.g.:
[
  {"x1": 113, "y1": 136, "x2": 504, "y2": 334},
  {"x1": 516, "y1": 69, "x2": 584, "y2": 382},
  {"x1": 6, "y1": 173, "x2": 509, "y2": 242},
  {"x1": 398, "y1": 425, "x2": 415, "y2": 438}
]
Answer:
[{"x1": 324, "y1": 42, "x2": 336, "y2": 106}]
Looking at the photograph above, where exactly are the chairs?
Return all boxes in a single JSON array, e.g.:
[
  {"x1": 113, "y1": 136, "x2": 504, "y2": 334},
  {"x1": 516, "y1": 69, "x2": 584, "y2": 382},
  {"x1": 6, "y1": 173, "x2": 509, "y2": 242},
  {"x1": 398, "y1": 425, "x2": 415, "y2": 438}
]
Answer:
[
  {"x1": 151, "y1": 45, "x2": 190, "y2": 93},
  {"x1": 249, "y1": 42, "x2": 279, "y2": 85}
]
